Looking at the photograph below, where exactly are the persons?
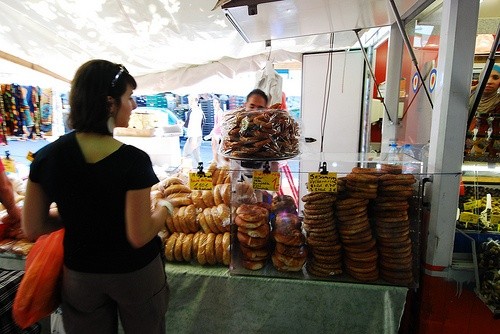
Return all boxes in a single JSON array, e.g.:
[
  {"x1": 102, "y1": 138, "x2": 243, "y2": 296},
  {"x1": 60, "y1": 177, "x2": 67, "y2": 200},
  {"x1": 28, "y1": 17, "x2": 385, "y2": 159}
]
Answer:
[
  {"x1": 469, "y1": 64, "x2": 500, "y2": 116},
  {"x1": 180, "y1": 94, "x2": 204, "y2": 162},
  {"x1": 217, "y1": 87, "x2": 287, "y2": 173},
  {"x1": 22, "y1": 60, "x2": 171, "y2": 334},
  {"x1": 208, "y1": 95, "x2": 224, "y2": 156},
  {"x1": 0, "y1": 156, "x2": 23, "y2": 222}
]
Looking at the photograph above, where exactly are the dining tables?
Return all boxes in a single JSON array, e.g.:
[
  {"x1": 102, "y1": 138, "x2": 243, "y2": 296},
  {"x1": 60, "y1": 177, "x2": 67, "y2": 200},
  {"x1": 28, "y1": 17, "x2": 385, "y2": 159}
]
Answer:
[{"x1": 162, "y1": 261, "x2": 409, "y2": 334}]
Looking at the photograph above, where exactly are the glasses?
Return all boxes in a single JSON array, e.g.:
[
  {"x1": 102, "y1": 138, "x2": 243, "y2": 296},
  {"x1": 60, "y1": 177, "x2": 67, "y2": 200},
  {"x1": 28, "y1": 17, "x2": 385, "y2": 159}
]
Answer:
[{"x1": 111, "y1": 63, "x2": 129, "y2": 88}]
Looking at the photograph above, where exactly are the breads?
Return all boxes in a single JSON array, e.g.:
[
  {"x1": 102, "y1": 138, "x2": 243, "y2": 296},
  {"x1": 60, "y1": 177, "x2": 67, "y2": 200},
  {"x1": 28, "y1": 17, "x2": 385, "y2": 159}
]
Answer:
[
  {"x1": 223, "y1": 103, "x2": 300, "y2": 158},
  {"x1": 0, "y1": 178, "x2": 57, "y2": 255},
  {"x1": 235, "y1": 195, "x2": 306, "y2": 271}
]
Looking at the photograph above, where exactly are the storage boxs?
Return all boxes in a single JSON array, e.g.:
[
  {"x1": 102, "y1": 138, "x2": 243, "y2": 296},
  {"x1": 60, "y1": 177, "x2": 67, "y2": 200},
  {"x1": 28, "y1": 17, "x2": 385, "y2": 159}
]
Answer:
[{"x1": 228, "y1": 154, "x2": 423, "y2": 288}]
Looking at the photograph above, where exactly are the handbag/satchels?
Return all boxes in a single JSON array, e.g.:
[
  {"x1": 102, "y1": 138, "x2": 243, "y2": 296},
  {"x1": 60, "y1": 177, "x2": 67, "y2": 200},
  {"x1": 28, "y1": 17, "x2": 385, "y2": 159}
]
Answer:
[{"x1": 13, "y1": 229, "x2": 65, "y2": 328}]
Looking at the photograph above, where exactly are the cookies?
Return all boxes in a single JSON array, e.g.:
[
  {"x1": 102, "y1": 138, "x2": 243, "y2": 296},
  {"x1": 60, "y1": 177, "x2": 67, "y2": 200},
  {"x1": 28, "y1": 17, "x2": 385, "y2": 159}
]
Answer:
[
  {"x1": 303, "y1": 164, "x2": 416, "y2": 282},
  {"x1": 150, "y1": 162, "x2": 258, "y2": 265}
]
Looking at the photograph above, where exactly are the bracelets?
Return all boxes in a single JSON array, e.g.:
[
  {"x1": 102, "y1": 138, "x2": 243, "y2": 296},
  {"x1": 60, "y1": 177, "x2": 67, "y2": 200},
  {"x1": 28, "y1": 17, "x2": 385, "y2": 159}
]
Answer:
[{"x1": 157, "y1": 199, "x2": 173, "y2": 216}]
